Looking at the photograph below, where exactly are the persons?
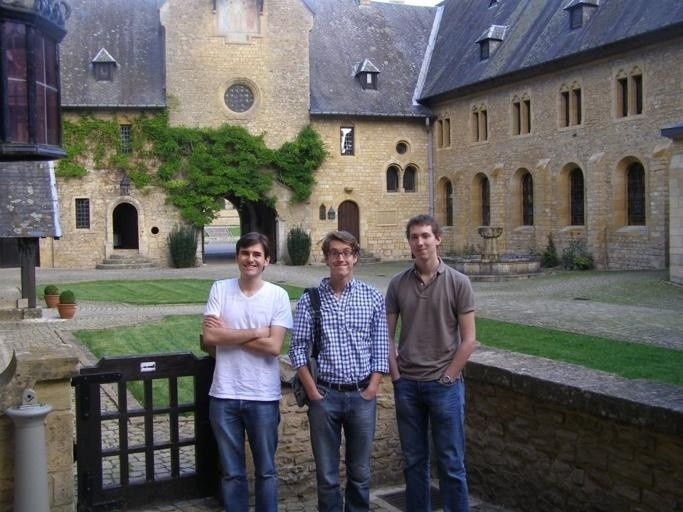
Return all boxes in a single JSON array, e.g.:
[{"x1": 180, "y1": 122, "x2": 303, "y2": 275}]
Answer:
[
  {"x1": 200, "y1": 232, "x2": 294, "y2": 511},
  {"x1": 291, "y1": 231, "x2": 393, "y2": 511},
  {"x1": 384, "y1": 215, "x2": 479, "y2": 512}
]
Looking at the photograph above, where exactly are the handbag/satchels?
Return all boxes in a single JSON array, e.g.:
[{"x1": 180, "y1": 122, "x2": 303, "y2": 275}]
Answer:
[{"x1": 291, "y1": 357, "x2": 321, "y2": 407}]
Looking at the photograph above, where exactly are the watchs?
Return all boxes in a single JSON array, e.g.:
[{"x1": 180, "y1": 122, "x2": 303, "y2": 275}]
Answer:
[{"x1": 442, "y1": 374, "x2": 454, "y2": 383}]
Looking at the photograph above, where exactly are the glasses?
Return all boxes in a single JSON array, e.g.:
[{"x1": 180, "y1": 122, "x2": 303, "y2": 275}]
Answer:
[{"x1": 327, "y1": 249, "x2": 353, "y2": 258}]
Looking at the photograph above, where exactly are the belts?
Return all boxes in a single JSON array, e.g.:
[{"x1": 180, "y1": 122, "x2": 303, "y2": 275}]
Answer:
[{"x1": 318, "y1": 378, "x2": 370, "y2": 393}]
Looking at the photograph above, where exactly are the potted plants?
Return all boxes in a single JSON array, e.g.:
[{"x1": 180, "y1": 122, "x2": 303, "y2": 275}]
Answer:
[{"x1": 43, "y1": 285, "x2": 77, "y2": 319}]
